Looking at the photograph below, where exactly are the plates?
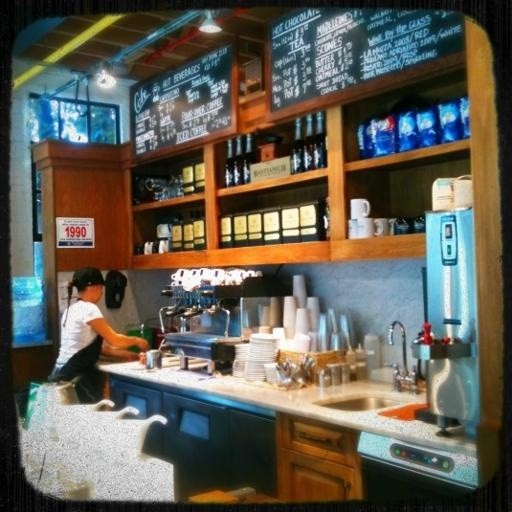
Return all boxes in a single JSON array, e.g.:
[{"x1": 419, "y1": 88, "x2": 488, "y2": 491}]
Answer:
[
  {"x1": 279, "y1": 336, "x2": 312, "y2": 353},
  {"x1": 259, "y1": 326, "x2": 272, "y2": 334},
  {"x1": 231, "y1": 344, "x2": 249, "y2": 378},
  {"x1": 263, "y1": 362, "x2": 297, "y2": 384},
  {"x1": 243, "y1": 333, "x2": 278, "y2": 382}
]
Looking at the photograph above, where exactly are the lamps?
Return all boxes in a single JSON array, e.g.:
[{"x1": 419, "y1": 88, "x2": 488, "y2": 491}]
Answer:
[
  {"x1": 199, "y1": 10, "x2": 222, "y2": 33},
  {"x1": 96, "y1": 61, "x2": 116, "y2": 89}
]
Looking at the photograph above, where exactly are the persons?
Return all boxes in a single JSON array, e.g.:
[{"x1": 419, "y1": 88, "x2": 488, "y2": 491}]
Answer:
[{"x1": 48, "y1": 267, "x2": 149, "y2": 404}]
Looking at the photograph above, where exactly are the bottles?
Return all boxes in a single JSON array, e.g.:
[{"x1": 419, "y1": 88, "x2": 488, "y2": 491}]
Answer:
[
  {"x1": 304, "y1": 113, "x2": 317, "y2": 171},
  {"x1": 244, "y1": 134, "x2": 255, "y2": 184},
  {"x1": 292, "y1": 117, "x2": 304, "y2": 174},
  {"x1": 225, "y1": 139, "x2": 236, "y2": 188},
  {"x1": 315, "y1": 111, "x2": 328, "y2": 170},
  {"x1": 234, "y1": 135, "x2": 245, "y2": 186}
]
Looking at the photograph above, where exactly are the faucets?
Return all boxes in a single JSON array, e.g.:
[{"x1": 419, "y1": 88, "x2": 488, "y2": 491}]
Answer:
[{"x1": 387, "y1": 320, "x2": 420, "y2": 395}]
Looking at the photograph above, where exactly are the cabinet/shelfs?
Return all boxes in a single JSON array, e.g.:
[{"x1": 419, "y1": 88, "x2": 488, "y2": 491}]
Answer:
[
  {"x1": 94, "y1": 334, "x2": 483, "y2": 500},
  {"x1": 31, "y1": 16, "x2": 503, "y2": 270}
]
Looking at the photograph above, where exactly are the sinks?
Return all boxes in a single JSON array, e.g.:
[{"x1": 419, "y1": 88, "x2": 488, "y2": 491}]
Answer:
[{"x1": 311, "y1": 395, "x2": 409, "y2": 413}]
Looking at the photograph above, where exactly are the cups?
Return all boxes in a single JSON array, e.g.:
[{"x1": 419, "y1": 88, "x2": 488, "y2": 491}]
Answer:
[
  {"x1": 347, "y1": 219, "x2": 358, "y2": 238},
  {"x1": 357, "y1": 217, "x2": 384, "y2": 239},
  {"x1": 318, "y1": 313, "x2": 330, "y2": 352},
  {"x1": 375, "y1": 218, "x2": 388, "y2": 235},
  {"x1": 331, "y1": 334, "x2": 344, "y2": 354},
  {"x1": 306, "y1": 296, "x2": 322, "y2": 333},
  {"x1": 269, "y1": 297, "x2": 281, "y2": 327},
  {"x1": 292, "y1": 273, "x2": 308, "y2": 308},
  {"x1": 295, "y1": 308, "x2": 308, "y2": 334},
  {"x1": 263, "y1": 306, "x2": 269, "y2": 325},
  {"x1": 335, "y1": 311, "x2": 351, "y2": 344},
  {"x1": 272, "y1": 327, "x2": 285, "y2": 344},
  {"x1": 282, "y1": 295, "x2": 297, "y2": 340},
  {"x1": 325, "y1": 305, "x2": 336, "y2": 338},
  {"x1": 349, "y1": 197, "x2": 371, "y2": 218}
]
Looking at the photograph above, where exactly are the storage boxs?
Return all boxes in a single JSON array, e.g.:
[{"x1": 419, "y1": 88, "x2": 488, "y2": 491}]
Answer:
[{"x1": 17, "y1": 366, "x2": 48, "y2": 389}]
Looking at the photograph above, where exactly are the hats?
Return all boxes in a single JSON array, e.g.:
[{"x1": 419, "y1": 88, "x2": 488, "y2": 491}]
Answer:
[{"x1": 74, "y1": 266, "x2": 108, "y2": 287}]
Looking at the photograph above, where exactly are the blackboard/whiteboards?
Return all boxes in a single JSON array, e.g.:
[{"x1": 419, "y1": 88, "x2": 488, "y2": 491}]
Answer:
[
  {"x1": 263, "y1": 5, "x2": 467, "y2": 124},
  {"x1": 129, "y1": 39, "x2": 236, "y2": 160}
]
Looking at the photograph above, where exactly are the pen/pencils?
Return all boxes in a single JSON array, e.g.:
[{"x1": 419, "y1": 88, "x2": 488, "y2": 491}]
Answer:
[{"x1": 275, "y1": 353, "x2": 315, "y2": 386}]
